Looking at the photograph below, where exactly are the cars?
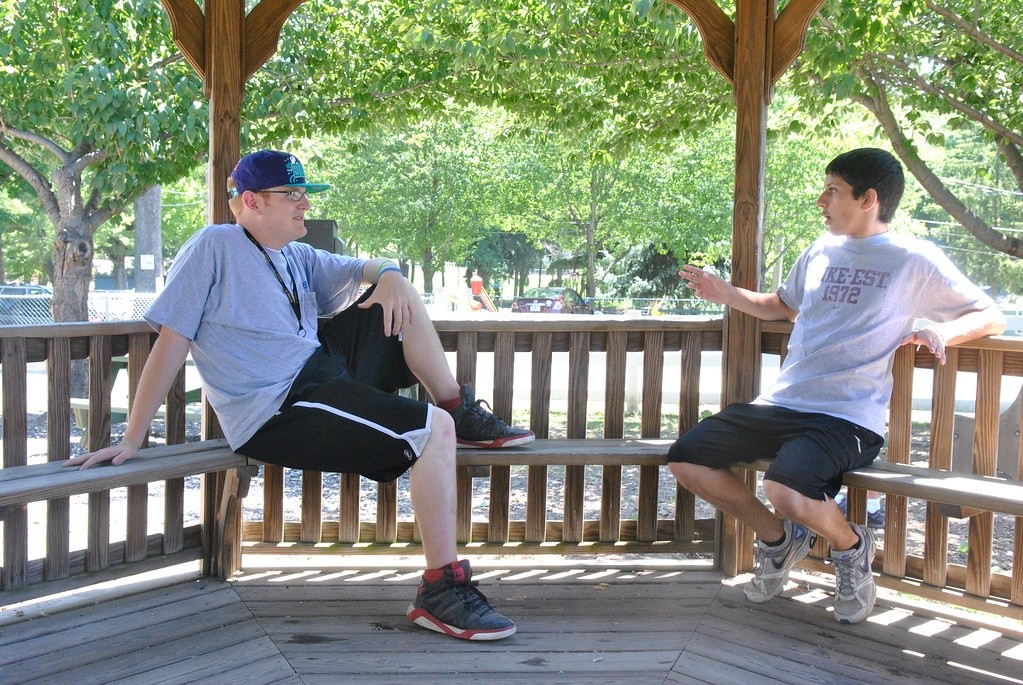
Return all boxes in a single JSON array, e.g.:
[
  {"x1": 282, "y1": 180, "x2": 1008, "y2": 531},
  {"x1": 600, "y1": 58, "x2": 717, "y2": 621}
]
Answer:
[
  {"x1": 510, "y1": 286, "x2": 596, "y2": 317},
  {"x1": 0, "y1": 281, "x2": 54, "y2": 317}
]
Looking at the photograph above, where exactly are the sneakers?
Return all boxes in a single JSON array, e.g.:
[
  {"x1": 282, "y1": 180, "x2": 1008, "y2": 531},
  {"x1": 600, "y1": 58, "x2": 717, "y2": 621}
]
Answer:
[
  {"x1": 437, "y1": 383, "x2": 535, "y2": 448},
  {"x1": 407, "y1": 560, "x2": 517, "y2": 640},
  {"x1": 823, "y1": 522, "x2": 877, "y2": 623},
  {"x1": 744, "y1": 517, "x2": 817, "y2": 604}
]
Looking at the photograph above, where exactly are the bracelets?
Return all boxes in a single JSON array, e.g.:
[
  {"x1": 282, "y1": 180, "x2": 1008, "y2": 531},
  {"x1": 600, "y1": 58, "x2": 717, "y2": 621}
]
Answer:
[{"x1": 376, "y1": 259, "x2": 403, "y2": 278}]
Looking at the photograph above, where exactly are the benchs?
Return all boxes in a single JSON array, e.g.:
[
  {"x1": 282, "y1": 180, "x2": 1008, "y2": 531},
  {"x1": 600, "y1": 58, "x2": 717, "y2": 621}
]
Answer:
[
  {"x1": 69, "y1": 397, "x2": 201, "y2": 418},
  {"x1": 0, "y1": 437, "x2": 1023, "y2": 519}
]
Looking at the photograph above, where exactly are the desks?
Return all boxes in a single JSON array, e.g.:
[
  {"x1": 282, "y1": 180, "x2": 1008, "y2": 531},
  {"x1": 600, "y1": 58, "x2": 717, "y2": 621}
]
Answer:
[{"x1": 78, "y1": 356, "x2": 203, "y2": 449}]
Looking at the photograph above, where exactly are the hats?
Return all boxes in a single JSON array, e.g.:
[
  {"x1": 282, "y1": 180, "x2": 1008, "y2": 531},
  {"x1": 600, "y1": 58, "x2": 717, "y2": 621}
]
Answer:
[{"x1": 227, "y1": 150, "x2": 330, "y2": 202}]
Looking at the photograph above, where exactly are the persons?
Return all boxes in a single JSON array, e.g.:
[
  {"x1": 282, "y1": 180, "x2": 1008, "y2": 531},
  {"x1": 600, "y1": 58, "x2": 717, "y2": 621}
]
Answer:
[
  {"x1": 666, "y1": 148, "x2": 1006, "y2": 624},
  {"x1": 62, "y1": 150, "x2": 536, "y2": 639}
]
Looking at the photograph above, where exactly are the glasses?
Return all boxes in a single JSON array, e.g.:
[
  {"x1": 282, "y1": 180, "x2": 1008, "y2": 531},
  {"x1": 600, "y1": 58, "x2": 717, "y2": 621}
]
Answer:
[{"x1": 240, "y1": 188, "x2": 308, "y2": 202}]
